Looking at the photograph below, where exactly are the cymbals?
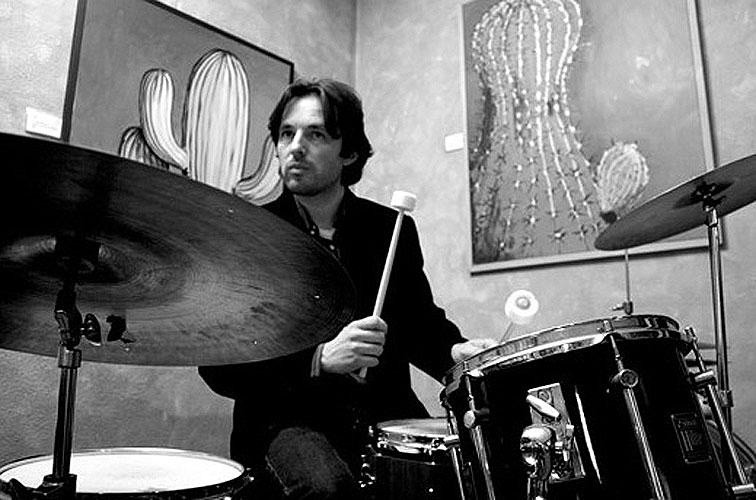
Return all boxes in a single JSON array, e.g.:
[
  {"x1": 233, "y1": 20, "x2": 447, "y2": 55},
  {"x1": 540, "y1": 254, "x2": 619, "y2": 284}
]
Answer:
[
  {"x1": 0, "y1": 132, "x2": 354, "y2": 369},
  {"x1": 593, "y1": 154, "x2": 756, "y2": 250}
]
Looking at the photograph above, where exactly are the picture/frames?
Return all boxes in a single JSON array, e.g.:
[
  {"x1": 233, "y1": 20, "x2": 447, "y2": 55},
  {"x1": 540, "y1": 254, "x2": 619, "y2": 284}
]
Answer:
[
  {"x1": 460, "y1": 0, "x2": 723, "y2": 273},
  {"x1": 61, "y1": 1, "x2": 295, "y2": 206}
]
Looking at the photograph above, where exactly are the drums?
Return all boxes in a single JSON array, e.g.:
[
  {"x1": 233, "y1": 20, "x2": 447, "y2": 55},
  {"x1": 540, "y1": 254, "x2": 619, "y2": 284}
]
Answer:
[
  {"x1": 0, "y1": 444, "x2": 253, "y2": 500},
  {"x1": 440, "y1": 315, "x2": 756, "y2": 500},
  {"x1": 362, "y1": 414, "x2": 459, "y2": 500}
]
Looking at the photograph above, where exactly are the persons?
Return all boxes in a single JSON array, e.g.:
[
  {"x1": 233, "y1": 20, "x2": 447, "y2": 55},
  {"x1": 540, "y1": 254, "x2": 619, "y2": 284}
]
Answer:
[{"x1": 198, "y1": 76, "x2": 500, "y2": 500}]
